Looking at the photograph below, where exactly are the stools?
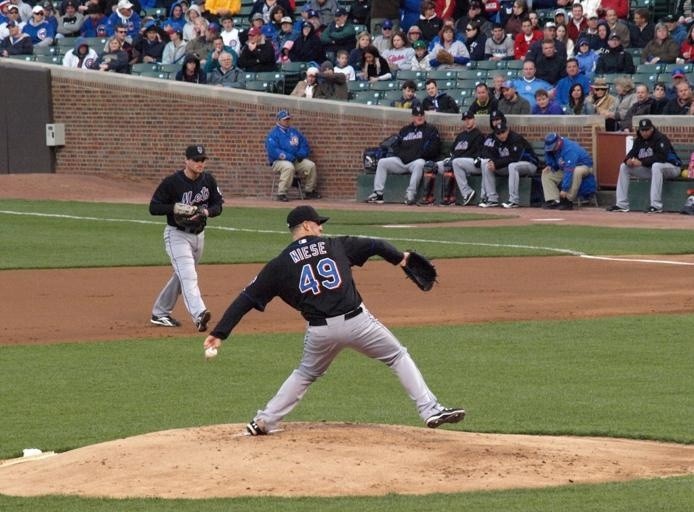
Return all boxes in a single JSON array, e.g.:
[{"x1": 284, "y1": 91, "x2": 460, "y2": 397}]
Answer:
[{"x1": 271, "y1": 175, "x2": 306, "y2": 200}]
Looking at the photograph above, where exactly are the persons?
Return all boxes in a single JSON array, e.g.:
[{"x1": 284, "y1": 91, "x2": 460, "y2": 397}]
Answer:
[
  {"x1": 0, "y1": 1, "x2": 693, "y2": 131},
  {"x1": 540, "y1": 131, "x2": 593, "y2": 208},
  {"x1": 361, "y1": 107, "x2": 439, "y2": 204},
  {"x1": 607, "y1": 119, "x2": 683, "y2": 213},
  {"x1": 450, "y1": 110, "x2": 505, "y2": 207},
  {"x1": 264, "y1": 110, "x2": 320, "y2": 202},
  {"x1": 204, "y1": 206, "x2": 465, "y2": 436},
  {"x1": 148, "y1": 145, "x2": 223, "y2": 332},
  {"x1": 417, "y1": 112, "x2": 484, "y2": 206},
  {"x1": 480, "y1": 125, "x2": 538, "y2": 208}
]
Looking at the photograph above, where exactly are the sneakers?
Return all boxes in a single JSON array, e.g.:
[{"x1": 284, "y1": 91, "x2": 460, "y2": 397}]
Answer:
[
  {"x1": 403, "y1": 191, "x2": 520, "y2": 208},
  {"x1": 644, "y1": 207, "x2": 663, "y2": 213},
  {"x1": 606, "y1": 205, "x2": 629, "y2": 212},
  {"x1": 361, "y1": 191, "x2": 384, "y2": 203},
  {"x1": 277, "y1": 194, "x2": 288, "y2": 201},
  {"x1": 151, "y1": 314, "x2": 182, "y2": 327},
  {"x1": 195, "y1": 310, "x2": 210, "y2": 332},
  {"x1": 277, "y1": 111, "x2": 291, "y2": 120},
  {"x1": 543, "y1": 199, "x2": 572, "y2": 210},
  {"x1": 306, "y1": 190, "x2": 320, "y2": 197},
  {"x1": 425, "y1": 408, "x2": 465, "y2": 428},
  {"x1": 247, "y1": 420, "x2": 267, "y2": 435}
]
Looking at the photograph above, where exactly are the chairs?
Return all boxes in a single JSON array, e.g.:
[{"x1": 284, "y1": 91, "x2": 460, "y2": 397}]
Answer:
[{"x1": 0, "y1": 1, "x2": 694, "y2": 114}]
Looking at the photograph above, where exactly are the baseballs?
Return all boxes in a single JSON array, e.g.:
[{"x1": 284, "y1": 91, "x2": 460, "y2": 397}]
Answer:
[{"x1": 205, "y1": 347, "x2": 218, "y2": 359}]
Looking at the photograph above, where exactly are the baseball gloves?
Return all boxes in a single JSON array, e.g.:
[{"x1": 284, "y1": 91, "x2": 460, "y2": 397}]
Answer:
[
  {"x1": 175, "y1": 205, "x2": 207, "y2": 227},
  {"x1": 401, "y1": 252, "x2": 437, "y2": 291}
]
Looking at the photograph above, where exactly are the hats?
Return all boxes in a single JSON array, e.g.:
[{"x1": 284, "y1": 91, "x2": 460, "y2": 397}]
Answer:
[
  {"x1": 287, "y1": 206, "x2": 329, "y2": 228},
  {"x1": 383, "y1": 20, "x2": 394, "y2": 30},
  {"x1": 610, "y1": 31, "x2": 621, "y2": 41},
  {"x1": 414, "y1": 40, "x2": 424, "y2": 48},
  {"x1": 308, "y1": 8, "x2": 346, "y2": 20},
  {"x1": 544, "y1": 132, "x2": 557, "y2": 151},
  {"x1": 248, "y1": 13, "x2": 293, "y2": 36},
  {"x1": 590, "y1": 79, "x2": 609, "y2": 88},
  {"x1": 639, "y1": 119, "x2": 651, "y2": 130},
  {"x1": 545, "y1": 11, "x2": 564, "y2": 27},
  {"x1": 186, "y1": 145, "x2": 209, "y2": 160},
  {"x1": 461, "y1": 111, "x2": 474, "y2": 120},
  {"x1": 118, "y1": 0, "x2": 133, "y2": 10},
  {"x1": 32, "y1": 5, "x2": 44, "y2": 13},
  {"x1": 412, "y1": 105, "x2": 423, "y2": 114},
  {"x1": 672, "y1": 68, "x2": 685, "y2": 78}
]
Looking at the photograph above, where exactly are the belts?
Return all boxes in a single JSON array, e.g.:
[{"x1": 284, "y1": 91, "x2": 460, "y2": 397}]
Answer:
[
  {"x1": 176, "y1": 227, "x2": 203, "y2": 234},
  {"x1": 309, "y1": 307, "x2": 362, "y2": 326}
]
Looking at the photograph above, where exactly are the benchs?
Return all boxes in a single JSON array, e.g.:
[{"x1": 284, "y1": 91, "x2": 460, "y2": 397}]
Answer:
[
  {"x1": 626, "y1": 144, "x2": 694, "y2": 211},
  {"x1": 355, "y1": 140, "x2": 546, "y2": 211}
]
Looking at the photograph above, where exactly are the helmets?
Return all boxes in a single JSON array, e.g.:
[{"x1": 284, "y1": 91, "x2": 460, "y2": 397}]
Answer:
[{"x1": 489, "y1": 110, "x2": 507, "y2": 133}]
